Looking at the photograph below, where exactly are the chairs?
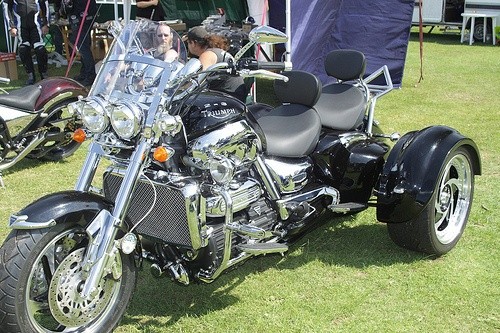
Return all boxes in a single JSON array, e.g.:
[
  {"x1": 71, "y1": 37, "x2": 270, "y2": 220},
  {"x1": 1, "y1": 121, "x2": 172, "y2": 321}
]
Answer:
[
  {"x1": 255, "y1": 71, "x2": 322, "y2": 160},
  {"x1": 308, "y1": 49, "x2": 365, "y2": 131}
]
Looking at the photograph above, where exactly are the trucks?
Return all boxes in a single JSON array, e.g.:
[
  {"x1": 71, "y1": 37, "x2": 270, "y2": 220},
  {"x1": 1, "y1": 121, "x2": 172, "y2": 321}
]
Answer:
[{"x1": 412, "y1": 0, "x2": 500, "y2": 43}]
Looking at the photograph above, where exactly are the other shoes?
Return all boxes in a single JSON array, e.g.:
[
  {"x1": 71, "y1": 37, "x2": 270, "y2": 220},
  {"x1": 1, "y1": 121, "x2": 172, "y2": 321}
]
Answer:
[
  {"x1": 75, "y1": 74, "x2": 82, "y2": 81},
  {"x1": 40, "y1": 72, "x2": 48, "y2": 80},
  {"x1": 27, "y1": 74, "x2": 36, "y2": 84},
  {"x1": 82, "y1": 80, "x2": 90, "y2": 86}
]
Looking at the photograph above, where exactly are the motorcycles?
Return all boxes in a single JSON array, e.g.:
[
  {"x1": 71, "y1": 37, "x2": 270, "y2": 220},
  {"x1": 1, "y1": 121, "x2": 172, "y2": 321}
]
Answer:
[
  {"x1": 0, "y1": 15, "x2": 482, "y2": 333},
  {"x1": 0, "y1": 75, "x2": 90, "y2": 172}
]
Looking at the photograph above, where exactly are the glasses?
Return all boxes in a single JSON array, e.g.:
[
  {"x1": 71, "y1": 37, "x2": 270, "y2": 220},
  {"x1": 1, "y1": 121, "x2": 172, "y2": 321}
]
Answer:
[{"x1": 158, "y1": 34, "x2": 168, "y2": 37}]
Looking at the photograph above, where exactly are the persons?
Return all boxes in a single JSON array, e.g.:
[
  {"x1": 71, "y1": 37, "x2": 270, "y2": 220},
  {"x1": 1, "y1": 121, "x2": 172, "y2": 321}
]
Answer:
[
  {"x1": 136, "y1": 0, "x2": 179, "y2": 21},
  {"x1": 48, "y1": 0, "x2": 77, "y2": 59},
  {"x1": 187, "y1": 27, "x2": 248, "y2": 105},
  {"x1": 60, "y1": 1, "x2": 98, "y2": 86},
  {"x1": 152, "y1": 24, "x2": 178, "y2": 64},
  {"x1": 3, "y1": 0, "x2": 50, "y2": 83}
]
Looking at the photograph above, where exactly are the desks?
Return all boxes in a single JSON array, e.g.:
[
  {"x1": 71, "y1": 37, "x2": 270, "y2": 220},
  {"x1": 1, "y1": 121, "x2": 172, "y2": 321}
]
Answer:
[
  {"x1": 45, "y1": 24, "x2": 80, "y2": 65},
  {"x1": 460, "y1": 13, "x2": 499, "y2": 46}
]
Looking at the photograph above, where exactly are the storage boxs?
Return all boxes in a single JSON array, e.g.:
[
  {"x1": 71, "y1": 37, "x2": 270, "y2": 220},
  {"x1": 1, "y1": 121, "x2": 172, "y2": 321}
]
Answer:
[{"x1": 0, "y1": 52, "x2": 18, "y2": 81}]
